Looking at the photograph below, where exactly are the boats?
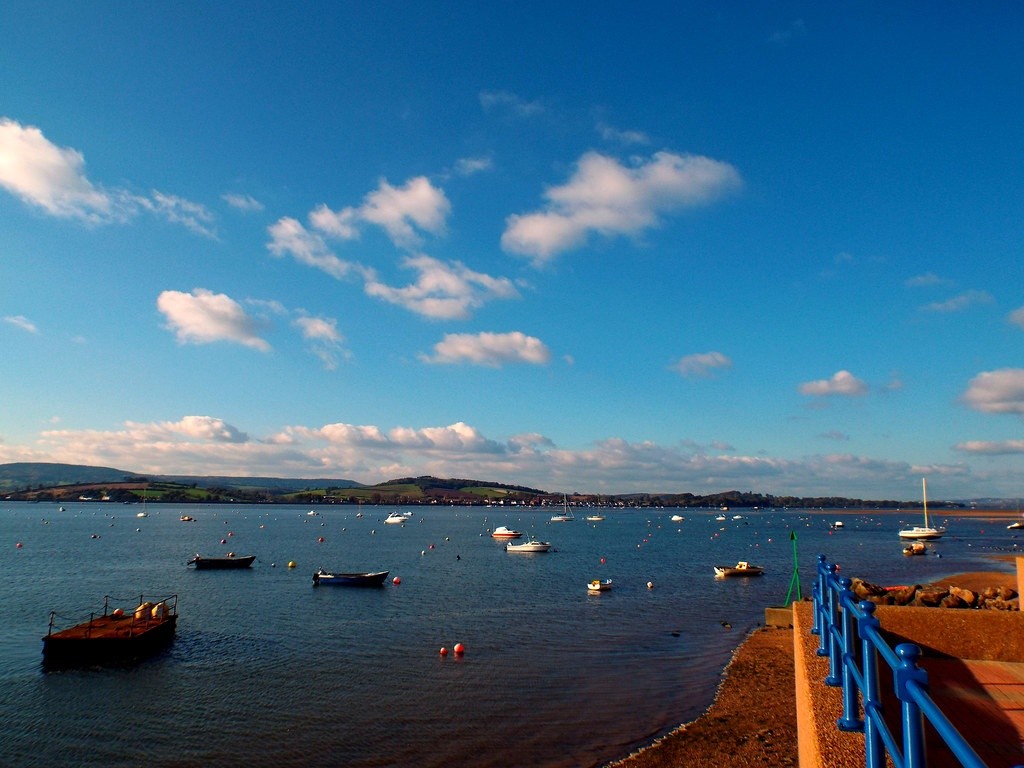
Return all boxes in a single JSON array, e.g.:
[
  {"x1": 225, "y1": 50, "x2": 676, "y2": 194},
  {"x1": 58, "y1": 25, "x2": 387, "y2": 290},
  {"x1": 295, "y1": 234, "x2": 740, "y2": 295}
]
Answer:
[
  {"x1": 403, "y1": 511, "x2": 415, "y2": 516},
  {"x1": 720, "y1": 506, "x2": 729, "y2": 511},
  {"x1": 586, "y1": 589, "x2": 602, "y2": 597},
  {"x1": 504, "y1": 539, "x2": 552, "y2": 552},
  {"x1": 38, "y1": 593, "x2": 178, "y2": 669},
  {"x1": 307, "y1": 510, "x2": 320, "y2": 516},
  {"x1": 491, "y1": 525, "x2": 524, "y2": 540},
  {"x1": 671, "y1": 515, "x2": 684, "y2": 522},
  {"x1": 384, "y1": 511, "x2": 409, "y2": 524},
  {"x1": 715, "y1": 516, "x2": 727, "y2": 521},
  {"x1": 733, "y1": 515, "x2": 744, "y2": 520},
  {"x1": 310, "y1": 565, "x2": 390, "y2": 587},
  {"x1": 179, "y1": 515, "x2": 193, "y2": 522},
  {"x1": 903, "y1": 540, "x2": 928, "y2": 556},
  {"x1": 832, "y1": 521, "x2": 846, "y2": 529},
  {"x1": 1007, "y1": 520, "x2": 1024, "y2": 530},
  {"x1": 186, "y1": 554, "x2": 258, "y2": 571},
  {"x1": 586, "y1": 579, "x2": 613, "y2": 590},
  {"x1": 714, "y1": 562, "x2": 765, "y2": 577}
]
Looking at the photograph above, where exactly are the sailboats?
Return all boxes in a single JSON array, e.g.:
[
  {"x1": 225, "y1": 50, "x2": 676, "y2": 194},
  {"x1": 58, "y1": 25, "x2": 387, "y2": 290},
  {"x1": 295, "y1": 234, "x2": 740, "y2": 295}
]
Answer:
[
  {"x1": 896, "y1": 477, "x2": 948, "y2": 541},
  {"x1": 136, "y1": 487, "x2": 148, "y2": 517},
  {"x1": 550, "y1": 493, "x2": 577, "y2": 522},
  {"x1": 586, "y1": 492, "x2": 606, "y2": 521}
]
